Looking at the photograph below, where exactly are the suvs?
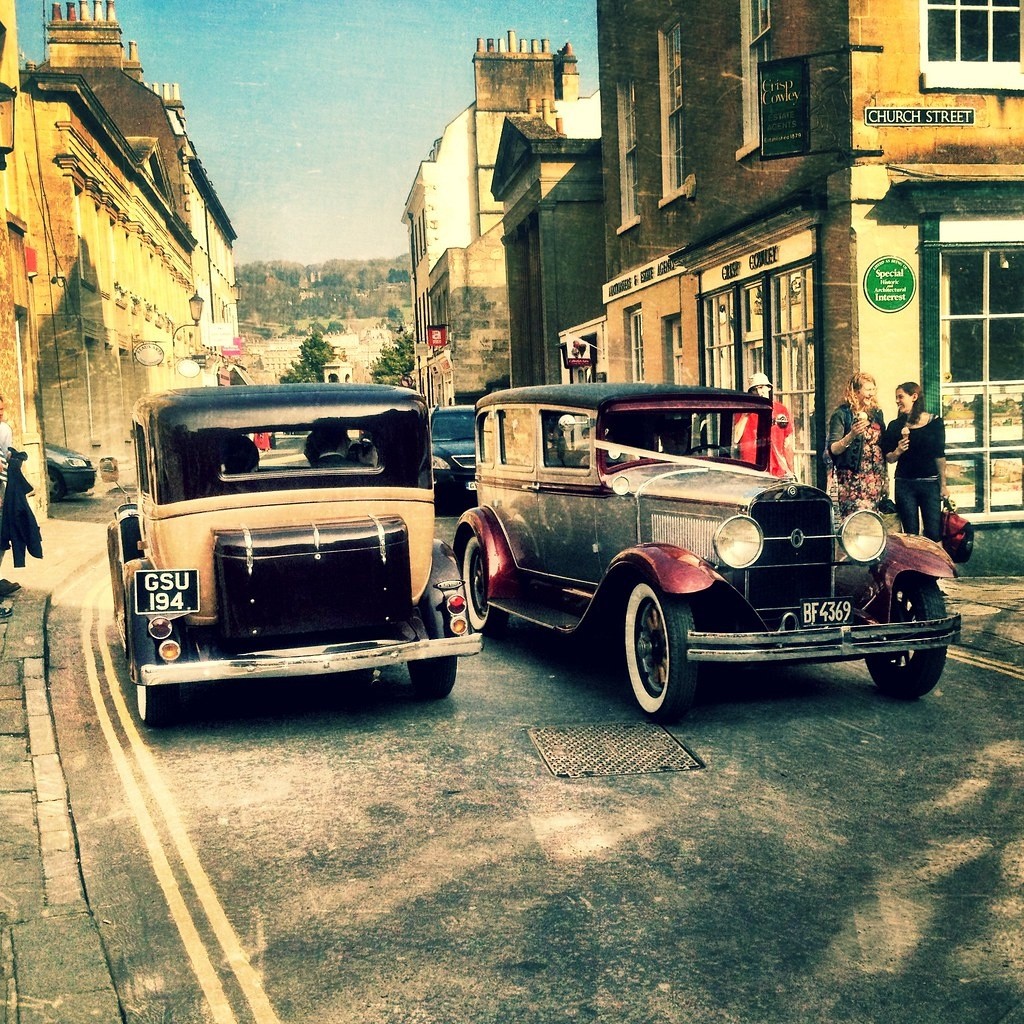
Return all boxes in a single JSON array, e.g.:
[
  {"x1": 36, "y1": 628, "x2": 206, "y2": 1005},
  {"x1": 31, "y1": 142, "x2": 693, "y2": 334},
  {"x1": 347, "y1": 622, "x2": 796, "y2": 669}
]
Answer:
[{"x1": 429, "y1": 404, "x2": 478, "y2": 517}]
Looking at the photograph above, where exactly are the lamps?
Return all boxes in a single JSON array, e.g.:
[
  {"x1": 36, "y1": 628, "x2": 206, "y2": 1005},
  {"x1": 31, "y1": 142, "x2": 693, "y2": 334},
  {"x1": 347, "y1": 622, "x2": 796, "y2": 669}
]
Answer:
[
  {"x1": 222, "y1": 280, "x2": 242, "y2": 309},
  {"x1": 173, "y1": 290, "x2": 204, "y2": 346}
]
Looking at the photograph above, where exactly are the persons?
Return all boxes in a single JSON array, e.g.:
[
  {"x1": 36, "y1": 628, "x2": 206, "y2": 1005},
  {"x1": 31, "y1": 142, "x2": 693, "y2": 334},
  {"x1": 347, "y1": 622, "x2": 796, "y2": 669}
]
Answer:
[
  {"x1": 564, "y1": 429, "x2": 640, "y2": 466},
  {"x1": 0, "y1": 396, "x2": 21, "y2": 619},
  {"x1": 882, "y1": 381, "x2": 951, "y2": 595},
  {"x1": 303, "y1": 424, "x2": 351, "y2": 469},
  {"x1": 733, "y1": 373, "x2": 795, "y2": 480},
  {"x1": 223, "y1": 435, "x2": 261, "y2": 473},
  {"x1": 827, "y1": 372, "x2": 890, "y2": 556}
]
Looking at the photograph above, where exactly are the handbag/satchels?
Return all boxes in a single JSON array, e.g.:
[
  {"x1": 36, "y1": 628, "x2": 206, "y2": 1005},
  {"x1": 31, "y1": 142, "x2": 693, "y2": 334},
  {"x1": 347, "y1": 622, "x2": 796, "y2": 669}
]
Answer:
[{"x1": 940, "y1": 497, "x2": 973, "y2": 563}]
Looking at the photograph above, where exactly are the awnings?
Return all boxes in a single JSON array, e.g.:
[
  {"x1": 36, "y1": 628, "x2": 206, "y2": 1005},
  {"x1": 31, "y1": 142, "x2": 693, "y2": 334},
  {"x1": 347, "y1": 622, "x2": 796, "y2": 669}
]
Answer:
[{"x1": 227, "y1": 362, "x2": 255, "y2": 385}]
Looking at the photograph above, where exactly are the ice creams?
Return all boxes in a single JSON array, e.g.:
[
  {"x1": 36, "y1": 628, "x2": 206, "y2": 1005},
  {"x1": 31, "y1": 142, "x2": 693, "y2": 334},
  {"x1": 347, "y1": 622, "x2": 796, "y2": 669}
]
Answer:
[
  {"x1": 901, "y1": 426, "x2": 910, "y2": 439},
  {"x1": 859, "y1": 412, "x2": 868, "y2": 424}
]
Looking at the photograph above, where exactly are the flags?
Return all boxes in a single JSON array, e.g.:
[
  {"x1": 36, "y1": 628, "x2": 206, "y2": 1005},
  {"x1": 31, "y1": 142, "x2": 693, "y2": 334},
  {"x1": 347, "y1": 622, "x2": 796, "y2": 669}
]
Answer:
[{"x1": 565, "y1": 333, "x2": 592, "y2": 366}]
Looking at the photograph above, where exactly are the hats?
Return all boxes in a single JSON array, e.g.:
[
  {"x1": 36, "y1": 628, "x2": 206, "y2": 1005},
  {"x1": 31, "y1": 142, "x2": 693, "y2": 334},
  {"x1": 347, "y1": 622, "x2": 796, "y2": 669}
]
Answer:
[{"x1": 747, "y1": 372, "x2": 773, "y2": 392}]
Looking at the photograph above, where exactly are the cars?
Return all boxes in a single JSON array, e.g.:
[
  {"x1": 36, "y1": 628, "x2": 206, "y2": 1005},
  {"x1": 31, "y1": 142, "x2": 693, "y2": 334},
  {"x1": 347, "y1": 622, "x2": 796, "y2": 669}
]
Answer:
[
  {"x1": 453, "y1": 382, "x2": 963, "y2": 724},
  {"x1": 44, "y1": 442, "x2": 95, "y2": 503},
  {"x1": 108, "y1": 383, "x2": 483, "y2": 729}
]
